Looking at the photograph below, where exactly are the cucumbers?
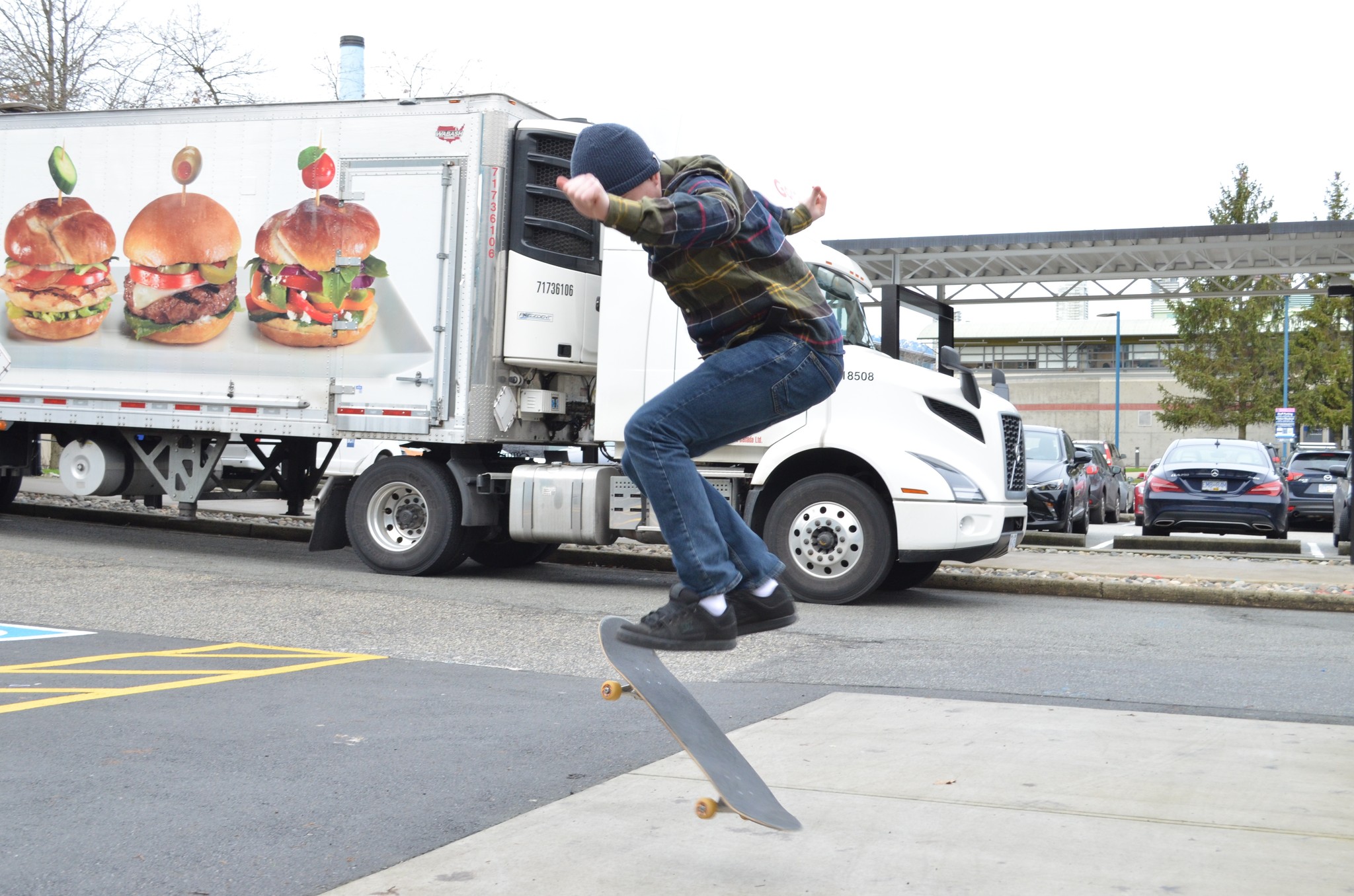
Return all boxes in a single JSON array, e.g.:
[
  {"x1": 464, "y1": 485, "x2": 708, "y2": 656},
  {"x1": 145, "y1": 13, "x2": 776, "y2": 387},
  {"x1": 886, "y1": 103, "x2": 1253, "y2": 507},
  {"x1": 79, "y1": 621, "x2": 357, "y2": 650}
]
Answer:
[{"x1": 48, "y1": 146, "x2": 77, "y2": 195}]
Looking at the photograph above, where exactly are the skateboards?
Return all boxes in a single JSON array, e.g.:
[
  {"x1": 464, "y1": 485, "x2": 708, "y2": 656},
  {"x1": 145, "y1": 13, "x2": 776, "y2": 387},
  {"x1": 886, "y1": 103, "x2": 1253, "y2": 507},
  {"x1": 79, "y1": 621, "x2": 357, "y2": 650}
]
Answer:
[{"x1": 594, "y1": 617, "x2": 805, "y2": 831}]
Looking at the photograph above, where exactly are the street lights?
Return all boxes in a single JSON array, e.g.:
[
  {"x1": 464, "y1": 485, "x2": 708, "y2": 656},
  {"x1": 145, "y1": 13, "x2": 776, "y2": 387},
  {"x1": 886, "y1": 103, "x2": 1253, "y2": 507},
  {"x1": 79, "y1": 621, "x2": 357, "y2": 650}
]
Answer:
[{"x1": 1097, "y1": 311, "x2": 1120, "y2": 454}]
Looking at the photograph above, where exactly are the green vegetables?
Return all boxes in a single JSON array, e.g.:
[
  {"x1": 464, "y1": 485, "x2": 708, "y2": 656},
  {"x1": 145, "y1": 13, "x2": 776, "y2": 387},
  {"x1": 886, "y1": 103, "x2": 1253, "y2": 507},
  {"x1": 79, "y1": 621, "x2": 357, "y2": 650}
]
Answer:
[{"x1": 297, "y1": 146, "x2": 327, "y2": 171}]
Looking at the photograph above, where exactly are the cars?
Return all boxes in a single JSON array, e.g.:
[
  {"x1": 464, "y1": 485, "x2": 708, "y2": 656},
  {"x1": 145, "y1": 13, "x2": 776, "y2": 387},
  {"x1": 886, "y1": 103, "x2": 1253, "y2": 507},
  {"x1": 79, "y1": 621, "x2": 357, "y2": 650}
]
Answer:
[
  {"x1": 1110, "y1": 466, "x2": 1135, "y2": 513},
  {"x1": 1072, "y1": 445, "x2": 1123, "y2": 524},
  {"x1": 1326, "y1": 453, "x2": 1353, "y2": 540},
  {"x1": 1027, "y1": 425, "x2": 1094, "y2": 534},
  {"x1": 1142, "y1": 437, "x2": 1289, "y2": 538},
  {"x1": 1134, "y1": 459, "x2": 1161, "y2": 526}
]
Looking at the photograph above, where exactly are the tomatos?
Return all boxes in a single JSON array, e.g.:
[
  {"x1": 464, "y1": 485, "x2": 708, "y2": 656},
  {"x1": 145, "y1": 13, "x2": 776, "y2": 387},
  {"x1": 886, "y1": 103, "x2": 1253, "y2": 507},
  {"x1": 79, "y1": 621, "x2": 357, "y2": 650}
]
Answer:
[{"x1": 301, "y1": 153, "x2": 335, "y2": 189}]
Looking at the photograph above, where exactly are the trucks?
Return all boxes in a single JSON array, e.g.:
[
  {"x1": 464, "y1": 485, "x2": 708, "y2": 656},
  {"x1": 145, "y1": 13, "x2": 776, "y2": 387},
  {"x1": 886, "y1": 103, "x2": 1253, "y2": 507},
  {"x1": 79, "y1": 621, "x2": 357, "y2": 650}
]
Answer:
[{"x1": 1, "y1": 91, "x2": 1027, "y2": 603}]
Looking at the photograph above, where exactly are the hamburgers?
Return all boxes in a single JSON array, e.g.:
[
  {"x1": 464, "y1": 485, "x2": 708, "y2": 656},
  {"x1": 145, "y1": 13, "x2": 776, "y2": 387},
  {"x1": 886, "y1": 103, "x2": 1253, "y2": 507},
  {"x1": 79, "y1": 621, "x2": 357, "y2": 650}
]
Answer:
[
  {"x1": 244, "y1": 194, "x2": 390, "y2": 348},
  {"x1": 122, "y1": 192, "x2": 242, "y2": 345},
  {"x1": 0, "y1": 196, "x2": 120, "y2": 340}
]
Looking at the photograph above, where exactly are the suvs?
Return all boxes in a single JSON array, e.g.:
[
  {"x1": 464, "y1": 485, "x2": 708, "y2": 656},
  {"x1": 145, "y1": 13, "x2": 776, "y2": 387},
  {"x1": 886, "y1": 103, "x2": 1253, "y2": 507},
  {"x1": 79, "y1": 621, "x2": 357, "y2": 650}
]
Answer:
[
  {"x1": 1265, "y1": 442, "x2": 1288, "y2": 472},
  {"x1": 1282, "y1": 441, "x2": 1351, "y2": 526},
  {"x1": 1072, "y1": 439, "x2": 1127, "y2": 474}
]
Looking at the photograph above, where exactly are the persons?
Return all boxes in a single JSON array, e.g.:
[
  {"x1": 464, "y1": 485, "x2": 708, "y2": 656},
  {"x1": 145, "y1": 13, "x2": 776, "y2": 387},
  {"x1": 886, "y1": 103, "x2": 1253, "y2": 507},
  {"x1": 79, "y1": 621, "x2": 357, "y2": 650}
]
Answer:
[{"x1": 552, "y1": 121, "x2": 848, "y2": 657}]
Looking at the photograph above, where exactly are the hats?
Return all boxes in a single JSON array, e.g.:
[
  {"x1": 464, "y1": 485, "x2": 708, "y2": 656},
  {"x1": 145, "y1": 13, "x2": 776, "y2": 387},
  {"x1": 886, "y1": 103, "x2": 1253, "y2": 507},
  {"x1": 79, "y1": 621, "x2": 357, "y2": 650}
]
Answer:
[{"x1": 570, "y1": 124, "x2": 661, "y2": 195}]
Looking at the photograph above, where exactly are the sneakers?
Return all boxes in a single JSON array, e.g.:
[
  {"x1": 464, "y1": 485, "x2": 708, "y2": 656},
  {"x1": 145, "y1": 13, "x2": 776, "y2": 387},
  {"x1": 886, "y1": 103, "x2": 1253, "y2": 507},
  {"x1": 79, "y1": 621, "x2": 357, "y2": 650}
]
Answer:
[
  {"x1": 724, "y1": 580, "x2": 797, "y2": 636},
  {"x1": 617, "y1": 583, "x2": 737, "y2": 652}
]
